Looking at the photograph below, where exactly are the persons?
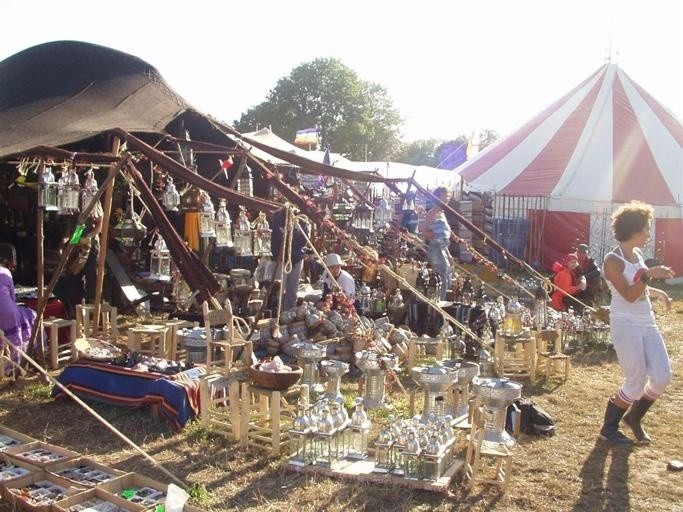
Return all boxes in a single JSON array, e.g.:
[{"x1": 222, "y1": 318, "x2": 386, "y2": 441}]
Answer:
[
  {"x1": 267, "y1": 186, "x2": 312, "y2": 318},
  {"x1": 551, "y1": 253, "x2": 593, "y2": 314},
  {"x1": 600, "y1": 202, "x2": 676, "y2": 446},
  {"x1": 570, "y1": 243, "x2": 601, "y2": 303},
  {"x1": 320, "y1": 253, "x2": 356, "y2": 304},
  {"x1": 421, "y1": 186, "x2": 458, "y2": 301},
  {"x1": 0, "y1": 241, "x2": 49, "y2": 377}
]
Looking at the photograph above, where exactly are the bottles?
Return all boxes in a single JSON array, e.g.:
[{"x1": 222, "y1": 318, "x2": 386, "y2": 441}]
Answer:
[
  {"x1": 150, "y1": 247, "x2": 171, "y2": 282},
  {"x1": 235, "y1": 162, "x2": 253, "y2": 195},
  {"x1": 163, "y1": 179, "x2": 276, "y2": 257},
  {"x1": 487, "y1": 294, "x2": 611, "y2": 344},
  {"x1": 39, "y1": 166, "x2": 103, "y2": 216},
  {"x1": 416, "y1": 269, "x2": 486, "y2": 309},
  {"x1": 357, "y1": 283, "x2": 402, "y2": 313}
]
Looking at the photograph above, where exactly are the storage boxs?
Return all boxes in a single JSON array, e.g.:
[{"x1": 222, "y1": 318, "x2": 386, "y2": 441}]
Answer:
[{"x1": 2, "y1": 472, "x2": 210, "y2": 512}]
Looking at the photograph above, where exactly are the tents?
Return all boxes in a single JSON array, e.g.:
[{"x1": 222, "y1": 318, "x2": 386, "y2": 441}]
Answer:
[{"x1": 450, "y1": 63, "x2": 683, "y2": 285}]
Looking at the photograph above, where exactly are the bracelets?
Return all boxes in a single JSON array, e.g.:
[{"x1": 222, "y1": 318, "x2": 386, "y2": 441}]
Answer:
[{"x1": 640, "y1": 272, "x2": 650, "y2": 284}]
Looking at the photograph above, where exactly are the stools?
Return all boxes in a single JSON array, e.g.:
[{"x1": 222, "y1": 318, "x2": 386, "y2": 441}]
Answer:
[{"x1": 0, "y1": 298, "x2": 570, "y2": 495}]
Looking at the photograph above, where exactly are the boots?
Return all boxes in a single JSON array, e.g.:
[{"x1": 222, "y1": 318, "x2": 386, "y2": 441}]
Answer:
[
  {"x1": 600, "y1": 396, "x2": 633, "y2": 445},
  {"x1": 624, "y1": 396, "x2": 655, "y2": 443}
]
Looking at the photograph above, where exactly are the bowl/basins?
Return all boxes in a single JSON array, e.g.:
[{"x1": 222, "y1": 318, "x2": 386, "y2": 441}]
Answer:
[{"x1": 247, "y1": 362, "x2": 304, "y2": 391}]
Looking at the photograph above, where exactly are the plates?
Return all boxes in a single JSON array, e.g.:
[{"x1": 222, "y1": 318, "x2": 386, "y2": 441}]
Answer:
[{"x1": 74, "y1": 336, "x2": 123, "y2": 363}]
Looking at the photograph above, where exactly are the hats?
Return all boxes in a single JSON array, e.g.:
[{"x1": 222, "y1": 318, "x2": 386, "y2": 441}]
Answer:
[
  {"x1": 577, "y1": 243, "x2": 589, "y2": 254},
  {"x1": 323, "y1": 252, "x2": 347, "y2": 268},
  {"x1": 565, "y1": 253, "x2": 578, "y2": 264}
]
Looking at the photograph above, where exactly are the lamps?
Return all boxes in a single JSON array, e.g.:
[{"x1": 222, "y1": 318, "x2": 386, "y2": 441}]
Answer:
[
  {"x1": 236, "y1": 160, "x2": 256, "y2": 198},
  {"x1": 160, "y1": 172, "x2": 180, "y2": 212},
  {"x1": 110, "y1": 186, "x2": 148, "y2": 249},
  {"x1": 141, "y1": 232, "x2": 175, "y2": 284},
  {"x1": 198, "y1": 191, "x2": 275, "y2": 257},
  {"x1": 36, "y1": 160, "x2": 104, "y2": 221},
  {"x1": 331, "y1": 177, "x2": 375, "y2": 231}
]
